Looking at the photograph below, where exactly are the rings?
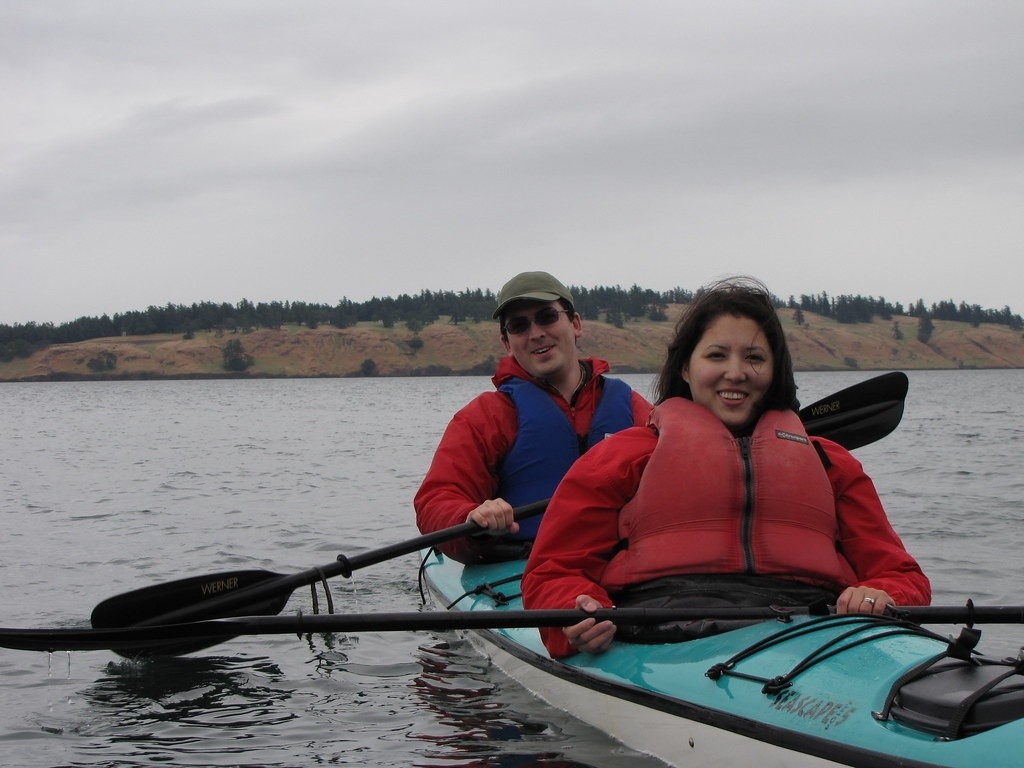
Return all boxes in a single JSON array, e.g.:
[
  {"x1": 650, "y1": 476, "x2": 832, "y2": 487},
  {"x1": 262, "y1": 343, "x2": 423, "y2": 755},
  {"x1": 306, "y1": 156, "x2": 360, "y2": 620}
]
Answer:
[{"x1": 864, "y1": 598, "x2": 875, "y2": 603}]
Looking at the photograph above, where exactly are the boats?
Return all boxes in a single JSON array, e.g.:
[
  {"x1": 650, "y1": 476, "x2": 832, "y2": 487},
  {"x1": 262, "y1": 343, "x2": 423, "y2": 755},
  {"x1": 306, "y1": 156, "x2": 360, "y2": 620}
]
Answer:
[{"x1": 416, "y1": 528, "x2": 1024, "y2": 768}]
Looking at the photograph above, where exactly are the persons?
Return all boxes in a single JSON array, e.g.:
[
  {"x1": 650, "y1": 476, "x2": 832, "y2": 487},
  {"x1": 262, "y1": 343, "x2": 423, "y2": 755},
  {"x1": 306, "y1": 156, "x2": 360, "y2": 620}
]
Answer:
[
  {"x1": 520, "y1": 276, "x2": 932, "y2": 660},
  {"x1": 414, "y1": 271, "x2": 655, "y2": 564}
]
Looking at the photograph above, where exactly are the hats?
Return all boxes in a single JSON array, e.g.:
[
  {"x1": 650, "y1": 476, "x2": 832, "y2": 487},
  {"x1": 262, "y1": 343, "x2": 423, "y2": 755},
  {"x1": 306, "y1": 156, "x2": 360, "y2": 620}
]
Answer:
[{"x1": 492, "y1": 271, "x2": 575, "y2": 320}]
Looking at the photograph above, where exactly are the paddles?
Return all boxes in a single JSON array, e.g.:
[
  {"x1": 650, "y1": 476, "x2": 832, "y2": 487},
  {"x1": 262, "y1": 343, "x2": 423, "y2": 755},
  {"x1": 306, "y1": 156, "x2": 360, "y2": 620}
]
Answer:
[
  {"x1": 89, "y1": 369, "x2": 909, "y2": 663},
  {"x1": 1, "y1": 593, "x2": 1023, "y2": 675}
]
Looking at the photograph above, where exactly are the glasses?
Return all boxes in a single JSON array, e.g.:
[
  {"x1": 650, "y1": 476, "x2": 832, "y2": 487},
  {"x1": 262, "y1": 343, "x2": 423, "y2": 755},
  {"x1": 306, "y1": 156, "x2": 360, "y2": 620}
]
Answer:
[{"x1": 502, "y1": 307, "x2": 568, "y2": 334}]
